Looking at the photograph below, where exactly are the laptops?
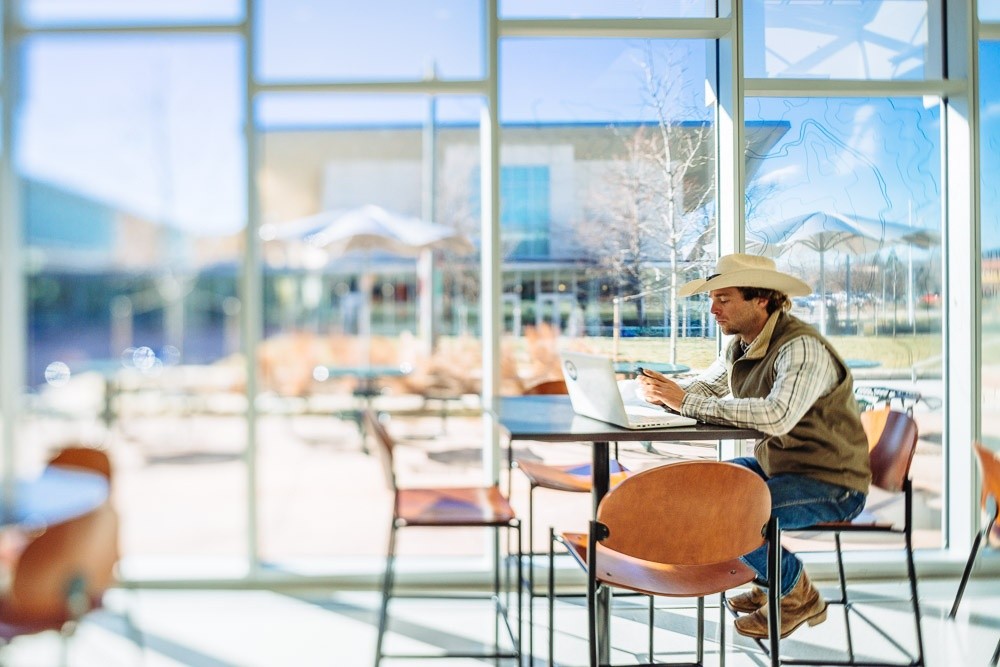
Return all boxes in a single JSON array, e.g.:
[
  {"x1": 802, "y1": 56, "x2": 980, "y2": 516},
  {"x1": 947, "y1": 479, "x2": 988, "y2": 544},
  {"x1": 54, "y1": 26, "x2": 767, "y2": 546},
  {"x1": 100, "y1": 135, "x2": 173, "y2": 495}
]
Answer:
[{"x1": 560, "y1": 351, "x2": 696, "y2": 429}]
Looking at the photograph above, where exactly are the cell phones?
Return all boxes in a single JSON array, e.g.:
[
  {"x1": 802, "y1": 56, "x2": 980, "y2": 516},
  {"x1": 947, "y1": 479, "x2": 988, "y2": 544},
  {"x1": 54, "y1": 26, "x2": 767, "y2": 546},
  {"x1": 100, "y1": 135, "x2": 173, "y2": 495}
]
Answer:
[{"x1": 634, "y1": 366, "x2": 651, "y2": 377}]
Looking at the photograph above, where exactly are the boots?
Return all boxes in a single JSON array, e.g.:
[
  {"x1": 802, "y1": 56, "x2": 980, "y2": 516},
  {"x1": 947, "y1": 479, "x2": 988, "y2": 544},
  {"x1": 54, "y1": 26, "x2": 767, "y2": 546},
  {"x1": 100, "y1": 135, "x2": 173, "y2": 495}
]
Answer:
[
  {"x1": 728, "y1": 584, "x2": 768, "y2": 613},
  {"x1": 734, "y1": 570, "x2": 825, "y2": 638}
]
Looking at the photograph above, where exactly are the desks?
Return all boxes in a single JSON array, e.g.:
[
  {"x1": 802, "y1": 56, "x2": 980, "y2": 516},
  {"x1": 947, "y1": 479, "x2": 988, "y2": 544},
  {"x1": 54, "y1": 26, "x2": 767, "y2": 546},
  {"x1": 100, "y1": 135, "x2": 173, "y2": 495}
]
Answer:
[{"x1": 483, "y1": 393, "x2": 772, "y2": 666}]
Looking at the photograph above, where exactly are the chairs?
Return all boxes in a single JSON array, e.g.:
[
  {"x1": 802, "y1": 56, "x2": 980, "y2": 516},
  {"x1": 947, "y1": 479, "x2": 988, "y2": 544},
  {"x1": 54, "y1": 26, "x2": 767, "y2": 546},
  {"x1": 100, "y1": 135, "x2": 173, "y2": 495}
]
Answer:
[
  {"x1": 0, "y1": 445, "x2": 129, "y2": 667},
  {"x1": 719, "y1": 412, "x2": 924, "y2": 667},
  {"x1": 234, "y1": 323, "x2": 647, "y2": 666},
  {"x1": 547, "y1": 459, "x2": 782, "y2": 667},
  {"x1": 945, "y1": 443, "x2": 1000, "y2": 667}
]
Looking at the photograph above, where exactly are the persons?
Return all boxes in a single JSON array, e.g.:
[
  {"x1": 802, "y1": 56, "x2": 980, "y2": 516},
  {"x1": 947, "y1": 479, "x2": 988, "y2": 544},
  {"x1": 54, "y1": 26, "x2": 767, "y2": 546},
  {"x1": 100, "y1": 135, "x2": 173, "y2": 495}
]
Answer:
[{"x1": 635, "y1": 253, "x2": 871, "y2": 640}]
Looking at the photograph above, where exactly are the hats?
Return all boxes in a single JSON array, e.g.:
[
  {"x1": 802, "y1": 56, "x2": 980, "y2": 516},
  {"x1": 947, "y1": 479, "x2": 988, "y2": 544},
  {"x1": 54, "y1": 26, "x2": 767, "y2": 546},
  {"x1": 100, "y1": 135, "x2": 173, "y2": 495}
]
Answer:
[{"x1": 679, "y1": 252, "x2": 811, "y2": 299}]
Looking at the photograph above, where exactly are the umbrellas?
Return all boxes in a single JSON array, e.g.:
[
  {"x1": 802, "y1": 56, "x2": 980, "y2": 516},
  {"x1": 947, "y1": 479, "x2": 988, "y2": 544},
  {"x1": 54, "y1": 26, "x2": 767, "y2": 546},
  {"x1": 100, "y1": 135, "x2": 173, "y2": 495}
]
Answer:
[
  {"x1": 272, "y1": 206, "x2": 471, "y2": 364},
  {"x1": 748, "y1": 211, "x2": 939, "y2": 333}
]
